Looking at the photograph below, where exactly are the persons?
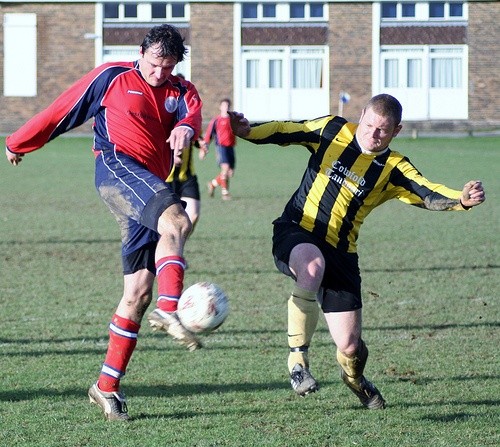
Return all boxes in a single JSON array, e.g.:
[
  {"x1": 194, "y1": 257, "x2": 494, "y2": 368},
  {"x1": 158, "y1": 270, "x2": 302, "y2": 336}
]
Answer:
[
  {"x1": 199, "y1": 98, "x2": 236, "y2": 202},
  {"x1": 4, "y1": 21, "x2": 209, "y2": 428},
  {"x1": 228, "y1": 92, "x2": 484, "y2": 410}
]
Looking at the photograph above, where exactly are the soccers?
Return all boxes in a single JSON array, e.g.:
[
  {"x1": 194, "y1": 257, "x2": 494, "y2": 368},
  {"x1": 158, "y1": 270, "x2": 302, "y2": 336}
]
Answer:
[{"x1": 178, "y1": 281, "x2": 229, "y2": 332}]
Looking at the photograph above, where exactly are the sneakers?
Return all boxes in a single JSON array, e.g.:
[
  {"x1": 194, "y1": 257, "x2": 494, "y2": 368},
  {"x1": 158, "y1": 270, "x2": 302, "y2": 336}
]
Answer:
[
  {"x1": 340, "y1": 368, "x2": 386, "y2": 410},
  {"x1": 207, "y1": 183, "x2": 216, "y2": 198},
  {"x1": 147, "y1": 308, "x2": 202, "y2": 353},
  {"x1": 87, "y1": 379, "x2": 130, "y2": 422},
  {"x1": 221, "y1": 189, "x2": 228, "y2": 195},
  {"x1": 288, "y1": 362, "x2": 320, "y2": 398}
]
